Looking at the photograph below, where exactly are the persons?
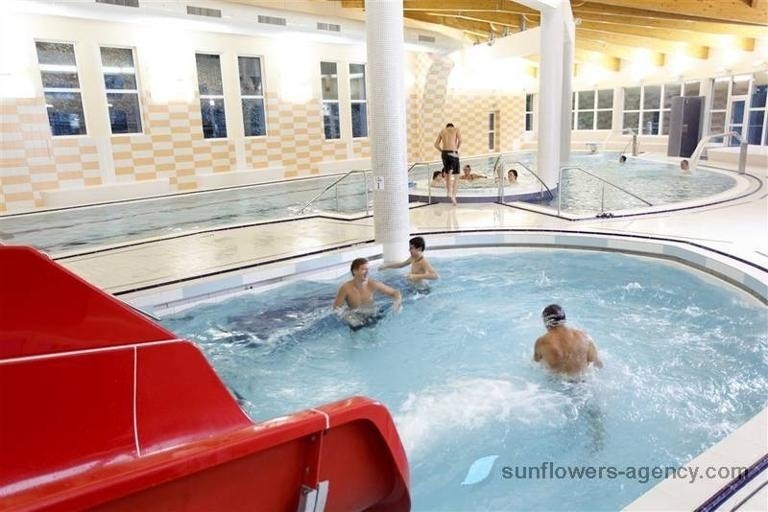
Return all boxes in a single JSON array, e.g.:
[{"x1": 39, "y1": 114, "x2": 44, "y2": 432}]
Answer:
[
  {"x1": 434, "y1": 122, "x2": 461, "y2": 205},
  {"x1": 333, "y1": 258, "x2": 403, "y2": 341},
  {"x1": 533, "y1": 304, "x2": 607, "y2": 454},
  {"x1": 440, "y1": 166, "x2": 456, "y2": 185},
  {"x1": 459, "y1": 164, "x2": 488, "y2": 181},
  {"x1": 378, "y1": 237, "x2": 440, "y2": 295},
  {"x1": 680, "y1": 160, "x2": 689, "y2": 172},
  {"x1": 502, "y1": 169, "x2": 519, "y2": 187},
  {"x1": 431, "y1": 172, "x2": 444, "y2": 188},
  {"x1": 619, "y1": 155, "x2": 627, "y2": 166}
]
